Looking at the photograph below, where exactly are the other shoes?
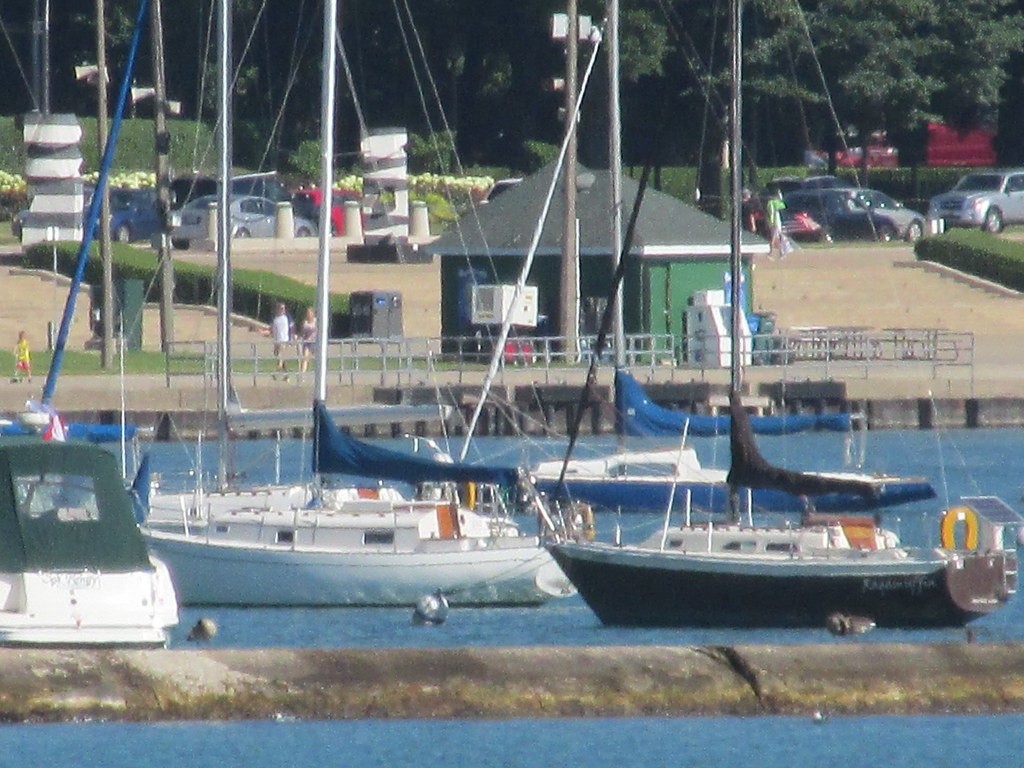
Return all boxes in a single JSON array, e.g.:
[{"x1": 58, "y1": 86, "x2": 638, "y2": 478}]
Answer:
[
  {"x1": 28, "y1": 377, "x2": 31, "y2": 383},
  {"x1": 780, "y1": 252, "x2": 787, "y2": 259},
  {"x1": 271, "y1": 371, "x2": 277, "y2": 380},
  {"x1": 766, "y1": 251, "x2": 775, "y2": 262},
  {"x1": 284, "y1": 374, "x2": 290, "y2": 381}
]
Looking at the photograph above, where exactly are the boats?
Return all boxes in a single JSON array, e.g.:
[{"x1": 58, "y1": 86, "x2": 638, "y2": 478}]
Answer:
[{"x1": 0, "y1": 418, "x2": 179, "y2": 654}]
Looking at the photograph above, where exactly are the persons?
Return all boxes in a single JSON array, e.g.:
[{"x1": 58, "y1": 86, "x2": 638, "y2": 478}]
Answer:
[
  {"x1": 256, "y1": 302, "x2": 296, "y2": 381},
  {"x1": 765, "y1": 182, "x2": 787, "y2": 262},
  {"x1": 10, "y1": 331, "x2": 32, "y2": 382},
  {"x1": 742, "y1": 188, "x2": 757, "y2": 232},
  {"x1": 297, "y1": 306, "x2": 321, "y2": 388}
]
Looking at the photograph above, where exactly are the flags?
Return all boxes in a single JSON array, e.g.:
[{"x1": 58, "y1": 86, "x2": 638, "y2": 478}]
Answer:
[{"x1": 42, "y1": 412, "x2": 69, "y2": 442}]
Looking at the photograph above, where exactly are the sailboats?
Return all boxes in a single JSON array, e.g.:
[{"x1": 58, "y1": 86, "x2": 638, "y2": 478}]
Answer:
[
  {"x1": 545, "y1": 0, "x2": 1017, "y2": 630},
  {"x1": 523, "y1": 0, "x2": 940, "y2": 509},
  {"x1": 109, "y1": 0, "x2": 591, "y2": 606}
]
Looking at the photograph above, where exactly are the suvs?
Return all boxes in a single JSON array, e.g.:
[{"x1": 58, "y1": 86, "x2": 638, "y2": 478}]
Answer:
[{"x1": 929, "y1": 172, "x2": 1024, "y2": 234}]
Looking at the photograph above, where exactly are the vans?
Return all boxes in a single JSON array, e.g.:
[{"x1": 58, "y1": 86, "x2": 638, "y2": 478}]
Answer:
[{"x1": 168, "y1": 167, "x2": 294, "y2": 218}]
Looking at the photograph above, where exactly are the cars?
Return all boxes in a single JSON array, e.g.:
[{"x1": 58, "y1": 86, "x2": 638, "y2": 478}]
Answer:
[
  {"x1": 783, "y1": 187, "x2": 926, "y2": 243},
  {"x1": 171, "y1": 193, "x2": 314, "y2": 249},
  {"x1": 11, "y1": 187, "x2": 168, "y2": 244},
  {"x1": 750, "y1": 175, "x2": 843, "y2": 219}
]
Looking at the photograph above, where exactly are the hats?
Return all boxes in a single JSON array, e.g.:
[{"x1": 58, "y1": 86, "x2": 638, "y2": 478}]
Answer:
[{"x1": 742, "y1": 189, "x2": 752, "y2": 194}]
